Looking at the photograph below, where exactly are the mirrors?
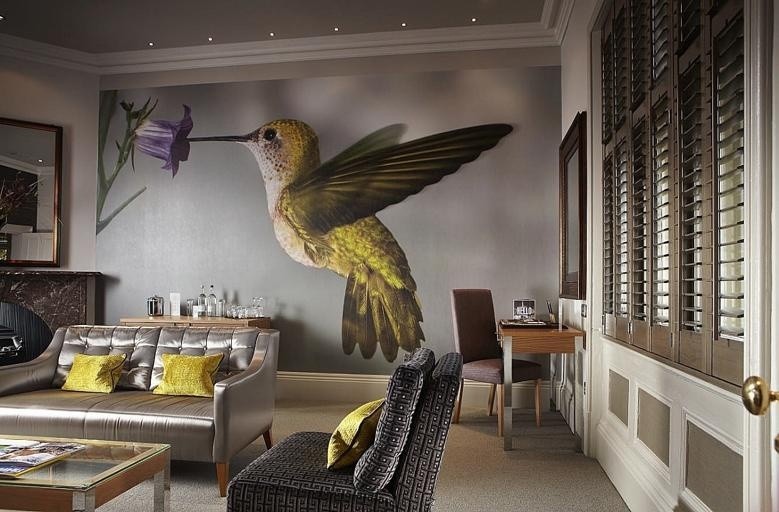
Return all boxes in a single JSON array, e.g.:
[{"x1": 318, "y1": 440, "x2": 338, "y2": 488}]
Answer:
[
  {"x1": 0, "y1": 116, "x2": 63, "y2": 267},
  {"x1": 559, "y1": 110, "x2": 586, "y2": 300}
]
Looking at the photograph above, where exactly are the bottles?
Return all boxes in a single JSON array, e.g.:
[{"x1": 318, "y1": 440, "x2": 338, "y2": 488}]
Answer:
[
  {"x1": 198, "y1": 284, "x2": 207, "y2": 316},
  {"x1": 206, "y1": 284, "x2": 216, "y2": 317}
]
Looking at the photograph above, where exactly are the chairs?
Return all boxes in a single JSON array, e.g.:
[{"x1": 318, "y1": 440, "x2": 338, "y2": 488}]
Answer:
[
  {"x1": 451, "y1": 288, "x2": 546, "y2": 435},
  {"x1": 225, "y1": 347, "x2": 464, "y2": 512}
]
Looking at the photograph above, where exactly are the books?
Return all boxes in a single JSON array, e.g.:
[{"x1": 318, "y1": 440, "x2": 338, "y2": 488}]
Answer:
[{"x1": 0, "y1": 438, "x2": 87, "y2": 478}]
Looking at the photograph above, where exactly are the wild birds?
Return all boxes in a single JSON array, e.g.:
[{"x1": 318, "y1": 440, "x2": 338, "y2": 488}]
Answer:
[{"x1": 175, "y1": 119, "x2": 513, "y2": 363}]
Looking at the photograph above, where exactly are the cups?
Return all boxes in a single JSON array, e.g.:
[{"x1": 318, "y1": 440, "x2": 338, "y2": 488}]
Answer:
[
  {"x1": 225, "y1": 295, "x2": 265, "y2": 318},
  {"x1": 193, "y1": 305, "x2": 198, "y2": 318},
  {"x1": 216, "y1": 299, "x2": 225, "y2": 317},
  {"x1": 185, "y1": 298, "x2": 195, "y2": 317}
]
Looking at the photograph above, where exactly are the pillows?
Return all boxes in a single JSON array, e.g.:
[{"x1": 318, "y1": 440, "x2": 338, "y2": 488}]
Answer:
[
  {"x1": 61, "y1": 352, "x2": 128, "y2": 393},
  {"x1": 352, "y1": 347, "x2": 435, "y2": 492},
  {"x1": 152, "y1": 352, "x2": 226, "y2": 397},
  {"x1": 326, "y1": 399, "x2": 385, "y2": 470}
]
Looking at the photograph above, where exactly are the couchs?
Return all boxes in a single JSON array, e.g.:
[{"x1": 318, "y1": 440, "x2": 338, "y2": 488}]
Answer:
[{"x1": 0, "y1": 324, "x2": 281, "y2": 497}]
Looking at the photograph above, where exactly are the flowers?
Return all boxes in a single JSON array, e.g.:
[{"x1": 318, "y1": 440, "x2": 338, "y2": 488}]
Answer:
[{"x1": 97, "y1": 89, "x2": 193, "y2": 236}]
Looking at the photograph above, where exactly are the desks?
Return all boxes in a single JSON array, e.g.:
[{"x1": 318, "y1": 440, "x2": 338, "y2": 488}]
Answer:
[
  {"x1": 499, "y1": 321, "x2": 585, "y2": 453},
  {"x1": 119, "y1": 314, "x2": 270, "y2": 329}
]
